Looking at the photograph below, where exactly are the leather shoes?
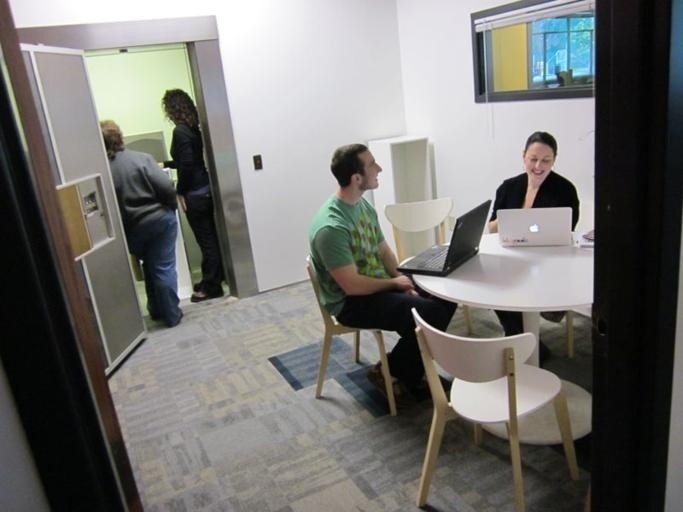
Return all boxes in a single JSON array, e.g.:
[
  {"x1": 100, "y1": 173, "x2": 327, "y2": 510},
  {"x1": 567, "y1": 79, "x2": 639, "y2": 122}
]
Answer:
[
  {"x1": 191, "y1": 292, "x2": 214, "y2": 302},
  {"x1": 194, "y1": 281, "x2": 204, "y2": 291}
]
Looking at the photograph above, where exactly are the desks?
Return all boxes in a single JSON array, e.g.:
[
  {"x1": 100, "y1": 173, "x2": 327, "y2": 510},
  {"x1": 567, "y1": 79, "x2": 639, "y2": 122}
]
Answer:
[{"x1": 409, "y1": 228, "x2": 593, "y2": 379}]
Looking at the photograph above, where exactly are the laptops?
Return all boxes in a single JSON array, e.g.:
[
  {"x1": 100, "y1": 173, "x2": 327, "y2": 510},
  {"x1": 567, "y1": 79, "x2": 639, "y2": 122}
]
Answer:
[
  {"x1": 496, "y1": 207, "x2": 574, "y2": 246},
  {"x1": 396, "y1": 199, "x2": 492, "y2": 276}
]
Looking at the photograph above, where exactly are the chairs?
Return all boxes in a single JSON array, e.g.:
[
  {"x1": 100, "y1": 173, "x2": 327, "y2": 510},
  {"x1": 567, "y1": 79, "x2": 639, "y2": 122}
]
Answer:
[
  {"x1": 407, "y1": 306, "x2": 586, "y2": 510},
  {"x1": 304, "y1": 252, "x2": 404, "y2": 418},
  {"x1": 383, "y1": 197, "x2": 474, "y2": 338}
]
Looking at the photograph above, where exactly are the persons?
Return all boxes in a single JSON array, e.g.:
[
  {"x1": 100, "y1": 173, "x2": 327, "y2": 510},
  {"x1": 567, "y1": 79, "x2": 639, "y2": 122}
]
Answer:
[
  {"x1": 309, "y1": 144, "x2": 457, "y2": 408},
  {"x1": 156, "y1": 88, "x2": 224, "y2": 302},
  {"x1": 488, "y1": 131, "x2": 579, "y2": 337},
  {"x1": 99, "y1": 120, "x2": 183, "y2": 327}
]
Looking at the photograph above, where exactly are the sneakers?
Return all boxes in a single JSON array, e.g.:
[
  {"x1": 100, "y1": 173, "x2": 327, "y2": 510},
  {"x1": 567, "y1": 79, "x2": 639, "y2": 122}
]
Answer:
[
  {"x1": 367, "y1": 356, "x2": 431, "y2": 416},
  {"x1": 151, "y1": 307, "x2": 183, "y2": 328},
  {"x1": 539, "y1": 341, "x2": 550, "y2": 367}
]
